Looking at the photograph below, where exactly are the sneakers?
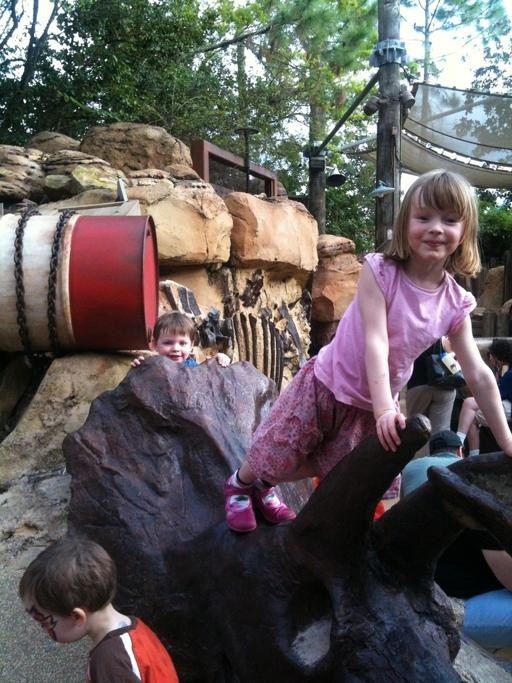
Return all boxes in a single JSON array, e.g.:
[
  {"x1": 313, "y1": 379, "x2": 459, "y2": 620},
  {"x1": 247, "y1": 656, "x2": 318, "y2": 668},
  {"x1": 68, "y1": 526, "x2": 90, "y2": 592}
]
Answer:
[
  {"x1": 256, "y1": 478, "x2": 296, "y2": 525},
  {"x1": 224, "y1": 467, "x2": 257, "y2": 533}
]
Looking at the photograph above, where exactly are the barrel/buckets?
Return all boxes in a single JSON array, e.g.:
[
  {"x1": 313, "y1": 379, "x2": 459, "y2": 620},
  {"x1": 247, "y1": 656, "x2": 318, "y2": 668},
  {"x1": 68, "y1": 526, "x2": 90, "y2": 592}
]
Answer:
[{"x1": 0, "y1": 212, "x2": 161, "y2": 351}]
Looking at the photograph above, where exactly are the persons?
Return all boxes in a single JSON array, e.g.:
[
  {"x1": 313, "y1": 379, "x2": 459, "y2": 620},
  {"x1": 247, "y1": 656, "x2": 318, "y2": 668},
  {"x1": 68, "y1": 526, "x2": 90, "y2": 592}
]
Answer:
[
  {"x1": 394, "y1": 332, "x2": 512, "y2": 648},
  {"x1": 129, "y1": 309, "x2": 231, "y2": 368},
  {"x1": 224, "y1": 167, "x2": 512, "y2": 533},
  {"x1": 18, "y1": 520, "x2": 181, "y2": 683}
]
